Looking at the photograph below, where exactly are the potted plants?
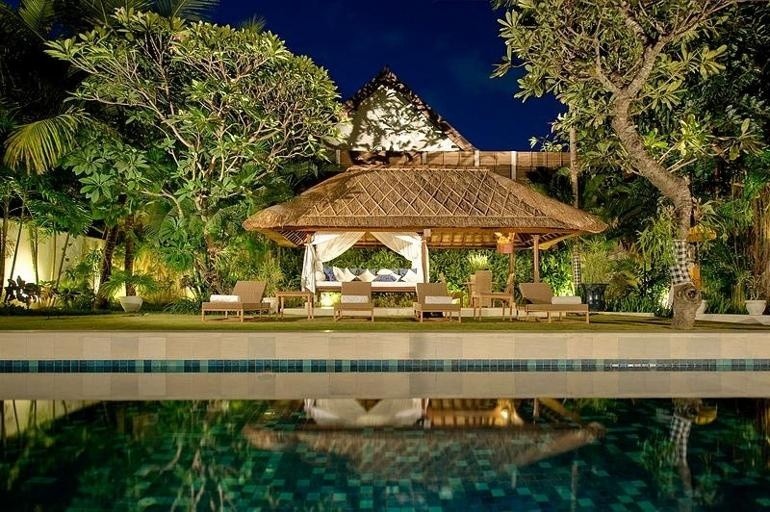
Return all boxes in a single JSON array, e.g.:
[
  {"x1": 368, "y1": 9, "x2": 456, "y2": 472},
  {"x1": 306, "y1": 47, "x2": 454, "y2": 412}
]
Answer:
[
  {"x1": 99, "y1": 266, "x2": 160, "y2": 313},
  {"x1": 736, "y1": 268, "x2": 768, "y2": 316}
]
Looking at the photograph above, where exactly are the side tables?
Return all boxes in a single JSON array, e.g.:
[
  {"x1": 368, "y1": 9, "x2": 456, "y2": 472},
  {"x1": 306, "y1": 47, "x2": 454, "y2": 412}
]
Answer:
[{"x1": 275, "y1": 292, "x2": 314, "y2": 320}]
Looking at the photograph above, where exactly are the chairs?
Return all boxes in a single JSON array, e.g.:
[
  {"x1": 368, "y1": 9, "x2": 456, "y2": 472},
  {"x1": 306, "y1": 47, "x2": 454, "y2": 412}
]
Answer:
[
  {"x1": 413, "y1": 283, "x2": 464, "y2": 324},
  {"x1": 467, "y1": 270, "x2": 515, "y2": 323},
  {"x1": 518, "y1": 283, "x2": 591, "y2": 326},
  {"x1": 200, "y1": 280, "x2": 269, "y2": 321},
  {"x1": 333, "y1": 281, "x2": 374, "y2": 322}
]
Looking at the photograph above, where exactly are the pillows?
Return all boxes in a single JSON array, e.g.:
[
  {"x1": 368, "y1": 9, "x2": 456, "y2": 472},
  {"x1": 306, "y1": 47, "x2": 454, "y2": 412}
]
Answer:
[
  {"x1": 340, "y1": 294, "x2": 370, "y2": 304},
  {"x1": 424, "y1": 295, "x2": 453, "y2": 304},
  {"x1": 551, "y1": 295, "x2": 583, "y2": 306},
  {"x1": 210, "y1": 293, "x2": 242, "y2": 303},
  {"x1": 314, "y1": 265, "x2": 418, "y2": 280}
]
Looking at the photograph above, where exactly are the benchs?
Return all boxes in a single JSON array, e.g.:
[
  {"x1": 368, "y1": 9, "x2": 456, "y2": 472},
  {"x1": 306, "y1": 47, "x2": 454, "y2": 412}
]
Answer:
[{"x1": 313, "y1": 267, "x2": 421, "y2": 307}]
